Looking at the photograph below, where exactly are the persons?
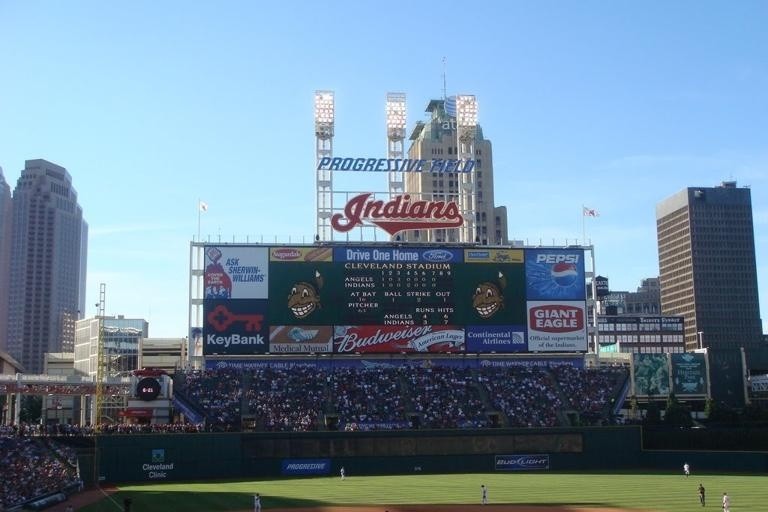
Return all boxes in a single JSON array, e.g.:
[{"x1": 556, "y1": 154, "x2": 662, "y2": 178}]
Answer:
[
  {"x1": 696, "y1": 482, "x2": 707, "y2": 506},
  {"x1": 683, "y1": 460, "x2": 690, "y2": 478},
  {"x1": 182, "y1": 362, "x2": 631, "y2": 431},
  {"x1": 721, "y1": 491, "x2": 731, "y2": 511},
  {"x1": 479, "y1": 484, "x2": 489, "y2": 504},
  {"x1": 0, "y1": 410, "x2": 107, "y2": 510},
  {"x1": 124, "y1": 495, "x2": 133, "y2": 512},
  {"x1": 340, "y1": 465, "x2": 345, "y2": 480},
  {"x1": 110, "y1": 421, "x2": 199, "y2": 434},
  {"x1": 253, "y1": 491, "x2": 261, "y2": 511}
]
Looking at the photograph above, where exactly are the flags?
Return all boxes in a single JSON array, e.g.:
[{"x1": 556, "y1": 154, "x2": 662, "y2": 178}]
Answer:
[
  {"x1": 198, "y1": 199, "x2": 211, "y2": 216},
  {"x1": 583, "y1": 206, "x2": 601, "y2": 219}
]
